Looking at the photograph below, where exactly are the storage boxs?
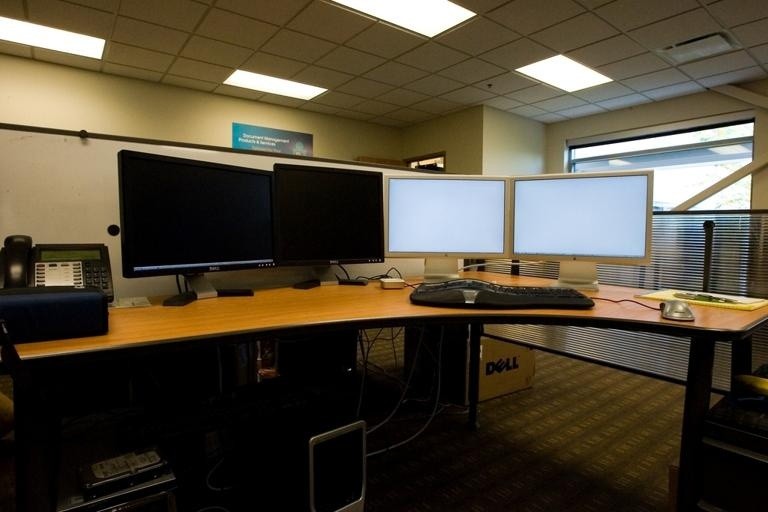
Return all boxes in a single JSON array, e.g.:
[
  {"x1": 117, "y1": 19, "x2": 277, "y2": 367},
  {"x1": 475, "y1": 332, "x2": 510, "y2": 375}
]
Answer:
[{"x1": 466, "y1": 340, "x2": 534, "y2": 403}]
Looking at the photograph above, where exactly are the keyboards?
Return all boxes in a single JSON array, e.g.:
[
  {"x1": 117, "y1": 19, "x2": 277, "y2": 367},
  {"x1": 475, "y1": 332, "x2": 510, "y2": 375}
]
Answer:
[{"x1": 410, "y1": 278, "x2": 595, "y2": 310}]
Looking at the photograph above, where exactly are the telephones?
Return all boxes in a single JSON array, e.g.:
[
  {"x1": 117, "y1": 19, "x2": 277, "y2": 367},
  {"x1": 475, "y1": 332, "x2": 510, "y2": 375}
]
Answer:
[{"x1": 0, "y1": 235, "x2": 114, "y2": 303}]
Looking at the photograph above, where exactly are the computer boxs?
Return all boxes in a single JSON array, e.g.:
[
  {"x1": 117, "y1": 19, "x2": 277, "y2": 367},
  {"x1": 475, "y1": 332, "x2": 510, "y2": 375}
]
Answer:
[{"x1": 238, "y1": 376, "x2": 368, "y2": 511}]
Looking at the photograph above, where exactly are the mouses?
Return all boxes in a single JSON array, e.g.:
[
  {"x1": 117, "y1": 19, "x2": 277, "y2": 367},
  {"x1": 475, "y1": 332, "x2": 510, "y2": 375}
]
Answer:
[{"x1": 659, "y1": 301, "x2": 695, "y2": 322}]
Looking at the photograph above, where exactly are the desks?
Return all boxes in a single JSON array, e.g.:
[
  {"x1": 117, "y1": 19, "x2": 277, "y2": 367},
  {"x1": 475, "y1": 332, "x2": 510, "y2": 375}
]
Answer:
[{"x1": 11, "y1": 269, "x2": 768, "y2": 511}]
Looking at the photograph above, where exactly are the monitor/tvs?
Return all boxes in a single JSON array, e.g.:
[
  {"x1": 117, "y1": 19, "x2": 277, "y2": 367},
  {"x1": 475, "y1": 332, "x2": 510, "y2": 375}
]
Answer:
[
  {"x1": 383, "y1": 174, "x2": 510, "y2": 282},
  {"x1": 274, "y1": 163, "x2": 384, "y2": 290},
  {"x1": 118, "y1": 150, "x2": 275, "y2": 306},
  {"x1": 510, "y1": 169, "x2": 653, "y2": 291}
]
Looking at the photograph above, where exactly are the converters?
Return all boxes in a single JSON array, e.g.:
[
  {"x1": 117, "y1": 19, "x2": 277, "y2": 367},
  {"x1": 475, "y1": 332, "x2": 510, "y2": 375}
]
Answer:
[{"x1": 380, "y1": 279, "x2": 405, "y2": 288}]
